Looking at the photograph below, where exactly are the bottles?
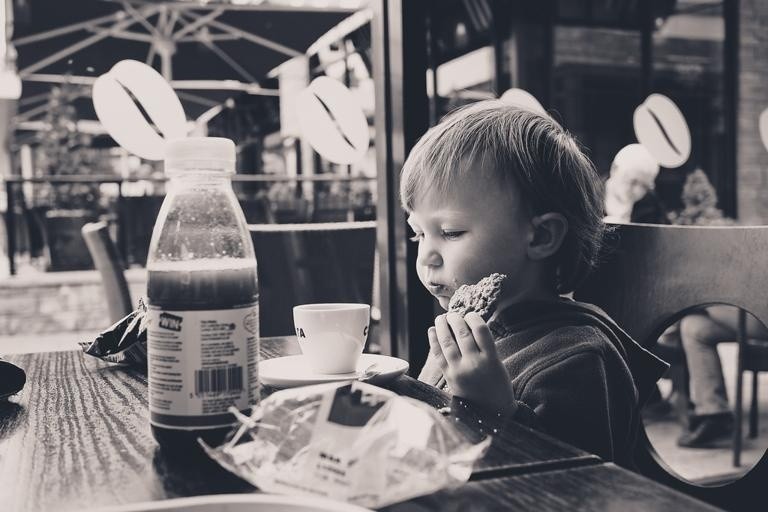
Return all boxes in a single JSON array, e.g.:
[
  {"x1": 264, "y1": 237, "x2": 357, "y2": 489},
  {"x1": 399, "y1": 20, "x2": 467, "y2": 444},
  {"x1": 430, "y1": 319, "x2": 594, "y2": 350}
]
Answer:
[{"x1": 144, "y1": 134, "x2": 263, "y2": 464}]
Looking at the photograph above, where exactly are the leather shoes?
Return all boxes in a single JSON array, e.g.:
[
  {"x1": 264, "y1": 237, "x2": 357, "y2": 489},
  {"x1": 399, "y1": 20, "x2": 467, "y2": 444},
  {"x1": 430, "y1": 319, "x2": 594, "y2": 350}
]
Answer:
[
  {"x1": 644, "y1": 398, "x2": 694, "y2": 418},
  {"x1": 677, "y1": 411, "x2": 737, "y2": 447}
]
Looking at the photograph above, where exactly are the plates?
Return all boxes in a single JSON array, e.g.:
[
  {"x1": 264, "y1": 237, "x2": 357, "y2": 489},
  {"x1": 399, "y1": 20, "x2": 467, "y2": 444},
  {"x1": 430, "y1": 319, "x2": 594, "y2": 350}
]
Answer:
[{"x1": 257, "y1": 352, "x2": 410, "y2": 388}]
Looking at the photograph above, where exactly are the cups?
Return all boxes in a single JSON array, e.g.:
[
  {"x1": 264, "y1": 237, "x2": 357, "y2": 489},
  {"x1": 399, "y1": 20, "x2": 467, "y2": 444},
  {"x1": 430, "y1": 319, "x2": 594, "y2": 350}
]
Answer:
[{"x1": 291, "y1": 301, "x2": 371, "y2": 374}]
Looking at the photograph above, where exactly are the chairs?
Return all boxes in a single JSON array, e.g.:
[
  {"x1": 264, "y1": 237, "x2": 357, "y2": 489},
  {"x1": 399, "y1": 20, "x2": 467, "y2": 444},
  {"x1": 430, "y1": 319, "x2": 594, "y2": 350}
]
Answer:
[{"x1": 546, "y1": 218, "x2": 768, "y2": 512}]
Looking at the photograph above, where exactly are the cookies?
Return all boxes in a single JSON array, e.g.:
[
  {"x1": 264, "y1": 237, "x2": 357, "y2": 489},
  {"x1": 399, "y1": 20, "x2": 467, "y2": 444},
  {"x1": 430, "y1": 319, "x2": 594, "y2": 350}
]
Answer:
[{"x1": 447, "y1": 272, "x2": 508, "y2": 323}]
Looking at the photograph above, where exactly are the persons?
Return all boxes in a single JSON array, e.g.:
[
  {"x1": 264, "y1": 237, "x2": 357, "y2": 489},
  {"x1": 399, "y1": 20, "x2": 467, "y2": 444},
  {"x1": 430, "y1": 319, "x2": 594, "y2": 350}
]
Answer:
[
  {"x1": 663, "y1": 302, "x2": 765, "y2": 448},
  {"x1": 399, "y1": 98, "x2": 670, "y2": 479},
  {"x1": 591, "y1": 140, "x2": 688, "y2": 419}
]
